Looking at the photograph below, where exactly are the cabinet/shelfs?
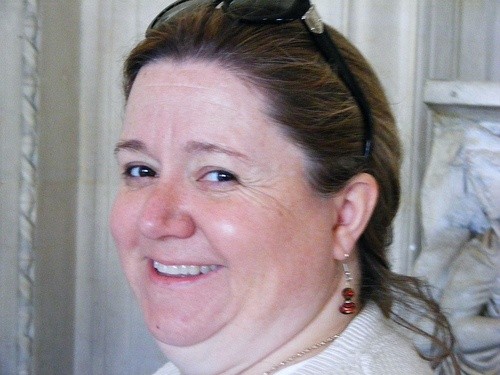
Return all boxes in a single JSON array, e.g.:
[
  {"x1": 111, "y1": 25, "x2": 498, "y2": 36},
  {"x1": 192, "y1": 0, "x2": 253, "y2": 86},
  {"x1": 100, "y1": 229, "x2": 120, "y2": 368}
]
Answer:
[{"x1": 394, "y1": 1, "x2": 500, "y2": 280}]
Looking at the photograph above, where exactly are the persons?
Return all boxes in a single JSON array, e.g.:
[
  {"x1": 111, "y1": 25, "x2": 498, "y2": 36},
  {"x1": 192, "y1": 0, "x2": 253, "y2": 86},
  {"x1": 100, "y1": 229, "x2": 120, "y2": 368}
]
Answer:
[
  {"x1": 110, "y1": 1, "x2": 459, "y2": 375},
  {"x1": 415, "y1": 150, "x2": 500, "y2": 375}
]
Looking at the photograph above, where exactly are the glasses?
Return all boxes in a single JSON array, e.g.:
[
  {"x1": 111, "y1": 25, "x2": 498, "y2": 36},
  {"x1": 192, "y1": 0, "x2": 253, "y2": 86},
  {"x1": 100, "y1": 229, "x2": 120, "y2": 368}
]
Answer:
[{"x1": 145, "y1": 0, "x2": 376, "y2": 170}]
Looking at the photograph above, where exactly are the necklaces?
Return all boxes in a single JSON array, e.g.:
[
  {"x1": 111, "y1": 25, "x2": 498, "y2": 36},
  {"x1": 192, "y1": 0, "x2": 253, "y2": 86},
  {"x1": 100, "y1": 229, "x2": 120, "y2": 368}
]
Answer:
[{"x1": 263, "y1": 334, "x2": 341, "y2": 375}]
